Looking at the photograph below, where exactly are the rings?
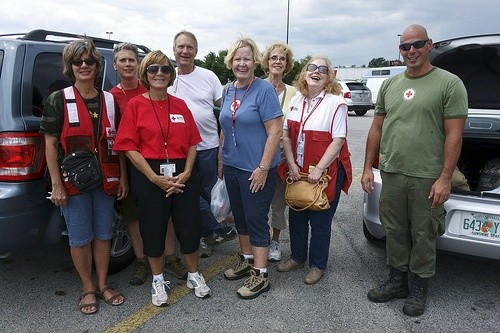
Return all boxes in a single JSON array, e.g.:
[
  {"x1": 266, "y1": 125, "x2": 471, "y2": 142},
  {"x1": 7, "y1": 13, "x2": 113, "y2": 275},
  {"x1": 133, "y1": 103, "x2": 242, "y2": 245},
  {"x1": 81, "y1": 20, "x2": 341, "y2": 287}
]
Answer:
[{"x1": 259, "y1": 184, "x2": 262, "y2": 186}]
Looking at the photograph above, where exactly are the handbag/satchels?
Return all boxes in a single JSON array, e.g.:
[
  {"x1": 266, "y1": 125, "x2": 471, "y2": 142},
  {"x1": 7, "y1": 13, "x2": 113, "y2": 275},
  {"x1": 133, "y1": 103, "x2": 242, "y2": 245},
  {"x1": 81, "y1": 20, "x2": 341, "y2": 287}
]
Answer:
[
  {"x1": 285, "y1": 172, "x2": 331, "y2": 212},
  {"x1": 61, "y1": 147, "x2": 104, "y2": 192},
  {"x1": 210, "y1": 174, "x2": 231, "y2": 223}
]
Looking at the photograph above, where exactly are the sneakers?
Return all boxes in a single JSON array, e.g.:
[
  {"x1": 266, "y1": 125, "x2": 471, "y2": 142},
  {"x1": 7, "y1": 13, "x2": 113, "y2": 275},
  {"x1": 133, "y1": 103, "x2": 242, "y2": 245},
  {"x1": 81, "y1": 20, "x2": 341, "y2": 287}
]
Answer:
[
  {"x1": 151, "y1": 276, "x2": 171, "y2": 307},
  {"x1": 236, "y1": 267, "x2": 270, "y2": 300},
  {"x1": 186, "y1": 271, "x2": 211, "y2": 298},
  {"x1": 225, "y1": 252, "x2": 254, "y2": 280}
]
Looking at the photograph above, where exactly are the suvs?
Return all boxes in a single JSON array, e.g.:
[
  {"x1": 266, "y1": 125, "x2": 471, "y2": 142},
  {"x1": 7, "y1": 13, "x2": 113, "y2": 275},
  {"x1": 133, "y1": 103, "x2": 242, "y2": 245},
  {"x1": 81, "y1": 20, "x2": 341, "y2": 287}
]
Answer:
[{"x1": 0, "y1": 29, "x2": 191, "y2": 275}]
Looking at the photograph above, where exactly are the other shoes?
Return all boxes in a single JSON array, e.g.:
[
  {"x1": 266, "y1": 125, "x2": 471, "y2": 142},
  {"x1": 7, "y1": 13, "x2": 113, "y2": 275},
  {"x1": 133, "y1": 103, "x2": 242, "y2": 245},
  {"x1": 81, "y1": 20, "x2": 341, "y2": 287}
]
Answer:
[
  {"x1": 304, "y1": 266, "x2": 323, "y2": 284},
  {"x1": 268, "y1": 240, "x2": 282, "y2": 261},
  {"x1": 164, "y1": 255, "x2": 187, "y2": 280},
  {"x1": 133, "y1": 256, "x2": 149, "y2": 286},
  {"x1": 277, "y1": 256, "x2": 304, "y2": 272}
]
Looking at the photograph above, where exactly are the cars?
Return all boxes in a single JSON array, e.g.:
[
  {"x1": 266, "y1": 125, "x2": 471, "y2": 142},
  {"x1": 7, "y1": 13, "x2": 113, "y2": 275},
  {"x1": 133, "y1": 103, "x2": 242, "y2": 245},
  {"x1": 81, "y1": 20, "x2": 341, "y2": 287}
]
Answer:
[
  {"x1": 335, "y1": 80, "x2": 374, "y2": 114},
  {"x1": 362, "y1": 33, "x2": 500, "y2": 260}
]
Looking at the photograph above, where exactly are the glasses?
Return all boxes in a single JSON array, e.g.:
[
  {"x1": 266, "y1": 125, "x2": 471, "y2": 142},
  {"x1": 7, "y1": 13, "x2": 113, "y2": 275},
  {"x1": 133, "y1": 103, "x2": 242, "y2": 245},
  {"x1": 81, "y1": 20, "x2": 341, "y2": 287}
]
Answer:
[
  {"x1": 306, "y1": 64, "x2": 328, "y2": 74},
  {"x1": 147, "y1": 65, "x2": 171, "y2": 74},
  {"x1": 400, "y1": 39, "x2": 428, "y2": 51},
  {"x1": 72, "y1": 57, "x2": 96, "y2": 66}
]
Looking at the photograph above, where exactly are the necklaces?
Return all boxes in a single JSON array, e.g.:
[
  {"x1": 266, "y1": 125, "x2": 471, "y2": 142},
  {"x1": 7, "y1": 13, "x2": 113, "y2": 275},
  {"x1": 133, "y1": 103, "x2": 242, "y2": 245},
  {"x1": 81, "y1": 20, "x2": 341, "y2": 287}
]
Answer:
[{"x1": 120, "y1": 79, "x2": 139, "y2": 101}]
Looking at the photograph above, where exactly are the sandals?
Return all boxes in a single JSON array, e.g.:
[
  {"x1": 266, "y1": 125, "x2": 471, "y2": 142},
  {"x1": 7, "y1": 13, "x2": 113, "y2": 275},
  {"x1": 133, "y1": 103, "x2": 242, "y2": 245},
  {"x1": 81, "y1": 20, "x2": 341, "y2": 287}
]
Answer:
[
  {"x1": 97, "y1": 284, "x2": 124, "y2": 306},
  {"x1": 79, "y1": 291, "x2": 99, "y2": 315}
]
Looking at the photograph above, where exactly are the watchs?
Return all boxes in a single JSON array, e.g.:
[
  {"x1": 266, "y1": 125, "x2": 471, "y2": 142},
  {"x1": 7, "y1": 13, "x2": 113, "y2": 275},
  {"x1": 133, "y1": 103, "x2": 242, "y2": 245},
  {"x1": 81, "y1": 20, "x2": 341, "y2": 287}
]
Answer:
[{"x1": 259, "y1": 165, "x2": 268, "y2": 171}]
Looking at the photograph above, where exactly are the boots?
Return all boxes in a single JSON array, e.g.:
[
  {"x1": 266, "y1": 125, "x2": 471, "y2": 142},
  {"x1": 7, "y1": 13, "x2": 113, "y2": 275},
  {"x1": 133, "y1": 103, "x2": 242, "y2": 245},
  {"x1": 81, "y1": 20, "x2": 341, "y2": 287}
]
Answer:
[
  {"x1": 403, "y1": 271, "x2": 428, "y2": 316},
  {"x1": 367, "y1": 265, "x2": 408, "y2": 302}
]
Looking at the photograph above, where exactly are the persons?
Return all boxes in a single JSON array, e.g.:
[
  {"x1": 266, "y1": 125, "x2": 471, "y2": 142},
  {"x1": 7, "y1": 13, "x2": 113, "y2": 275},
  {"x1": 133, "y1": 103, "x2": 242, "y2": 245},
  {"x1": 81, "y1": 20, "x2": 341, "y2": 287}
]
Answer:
[
  {"x1": 360, "y1": 23, "x2": 468, "y2": 316},
  {"x1": 277, "y1": 56, "x2": 352, "y2": 283},
  {"x1": 218, "y1": 41, "x2": 284, "y2": 299},
  {"x1": 106, "y1": 42, "x2": 188, "y2": 285},
  {"x1": 39, "y1": 38, "x2": 129, "y2": 315},
  {"x1": 166, "y1": 31, "x2": 238, "y2": 257},
  {"x1": 262, "y1": 43, "x2": 297, "y2": 261},
  {"x1": 114, "y1": 50, "x2": 212, "y2": 307}
]
